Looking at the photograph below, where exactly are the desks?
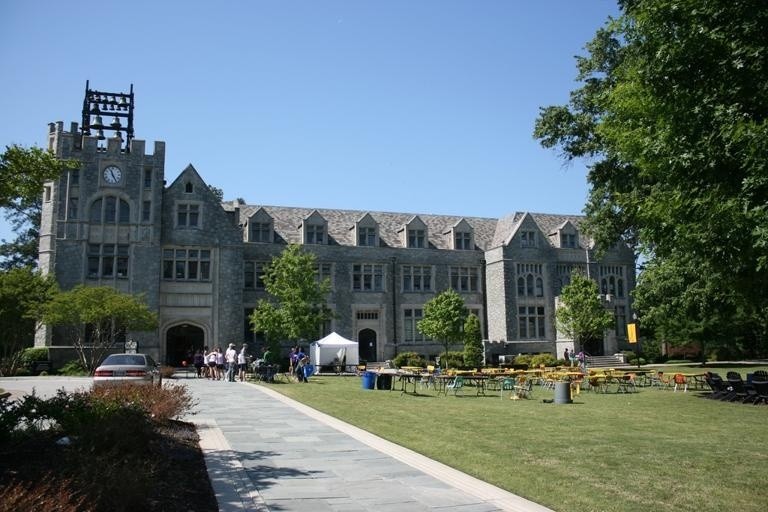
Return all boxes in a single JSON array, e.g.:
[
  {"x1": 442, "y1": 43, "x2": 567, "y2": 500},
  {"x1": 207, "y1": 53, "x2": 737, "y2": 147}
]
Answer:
[{"x1": 243, "y1": 361, "x2": 360, "y2": 383}]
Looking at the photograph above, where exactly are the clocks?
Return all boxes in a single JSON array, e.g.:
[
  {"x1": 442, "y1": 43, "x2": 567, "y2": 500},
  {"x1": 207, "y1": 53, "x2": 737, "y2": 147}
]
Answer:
[{"x1": 103, "y1": 164, "x2": 122, "y2": 184}]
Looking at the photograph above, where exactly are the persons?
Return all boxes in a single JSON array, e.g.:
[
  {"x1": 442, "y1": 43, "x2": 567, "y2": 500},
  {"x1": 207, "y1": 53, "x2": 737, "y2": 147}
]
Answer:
[
  {"x1": 186, "y1": 342, "x2": 313, "y2": 383},
  {"x1": 563, "y1": 348, "x2": 569, "y2": 362},
  {"x1": 577, "y1": 349, "x2": 585, "y2": 368},
  {"x1": 568, "y1": 349, "x2": 574, "y2": 358}
]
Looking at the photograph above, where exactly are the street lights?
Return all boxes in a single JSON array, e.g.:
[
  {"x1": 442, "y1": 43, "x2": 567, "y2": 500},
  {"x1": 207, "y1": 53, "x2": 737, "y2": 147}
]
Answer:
[{"x1": 631, "y1": 311, "x2": 640, "y2": 368}]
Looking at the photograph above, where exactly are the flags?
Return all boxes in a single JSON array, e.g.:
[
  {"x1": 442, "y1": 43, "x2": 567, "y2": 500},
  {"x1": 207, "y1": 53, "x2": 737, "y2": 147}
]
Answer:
[{"x1": 627, "y1": 323, "x2": 637, "y2": 343}]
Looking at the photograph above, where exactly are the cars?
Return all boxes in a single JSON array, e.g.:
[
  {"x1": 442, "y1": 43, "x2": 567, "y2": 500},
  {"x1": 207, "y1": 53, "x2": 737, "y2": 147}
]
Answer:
[{"x1": 93, "y1": 353, "x2": 163, "y2": 391}]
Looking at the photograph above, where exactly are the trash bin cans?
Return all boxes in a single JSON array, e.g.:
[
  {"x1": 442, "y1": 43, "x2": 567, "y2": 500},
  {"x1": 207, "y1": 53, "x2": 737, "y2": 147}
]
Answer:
[
  {"x1": 555, "y1": 382, "x2": 573, "y2": 403},
  {"x1": 362, "y1": 371, "x2": 376, "y2": 389},
  {"x1": 377, "y1": 374, "x2": 392, "y2": 389}
]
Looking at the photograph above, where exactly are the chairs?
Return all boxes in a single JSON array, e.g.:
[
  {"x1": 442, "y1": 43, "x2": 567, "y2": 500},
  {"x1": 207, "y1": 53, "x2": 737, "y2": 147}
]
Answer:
[{"x1": 360, "y1": 361, "x2": 768, "y2": 406}]
